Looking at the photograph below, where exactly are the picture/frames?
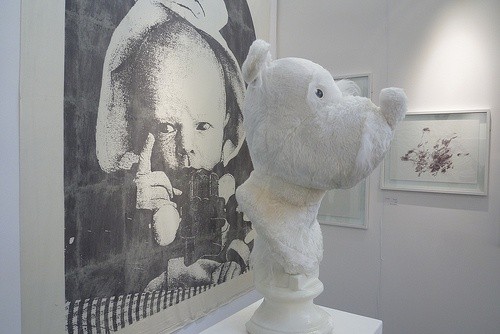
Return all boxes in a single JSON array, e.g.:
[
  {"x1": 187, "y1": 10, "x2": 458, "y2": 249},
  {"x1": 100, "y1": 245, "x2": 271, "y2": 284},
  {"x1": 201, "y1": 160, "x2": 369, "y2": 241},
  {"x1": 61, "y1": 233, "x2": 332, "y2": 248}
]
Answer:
[
  {"x1": 21, "y1": 0, "x2": 276, "y2": 334},
  {"x1": 318, "y1": 72, "x2": 369, "y2": 230},
  {"x1": 379, "y1": 108, "x2": 491, "y2": 198}
]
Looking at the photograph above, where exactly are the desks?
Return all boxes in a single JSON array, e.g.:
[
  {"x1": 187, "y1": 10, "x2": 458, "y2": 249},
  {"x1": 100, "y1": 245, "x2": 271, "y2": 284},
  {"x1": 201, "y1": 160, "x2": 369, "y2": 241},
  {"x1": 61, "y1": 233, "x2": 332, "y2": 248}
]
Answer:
[{"x1": 202, "y1": 290, "x2": 382, "y2": 334}]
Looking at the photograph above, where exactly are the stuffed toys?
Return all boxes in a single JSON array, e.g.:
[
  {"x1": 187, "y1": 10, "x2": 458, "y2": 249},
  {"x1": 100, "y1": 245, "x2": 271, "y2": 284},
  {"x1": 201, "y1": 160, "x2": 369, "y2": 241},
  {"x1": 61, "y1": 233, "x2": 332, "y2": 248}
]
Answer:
[{"x1": 236, "y1": 38, "x2": 409, "y2": 276}]
